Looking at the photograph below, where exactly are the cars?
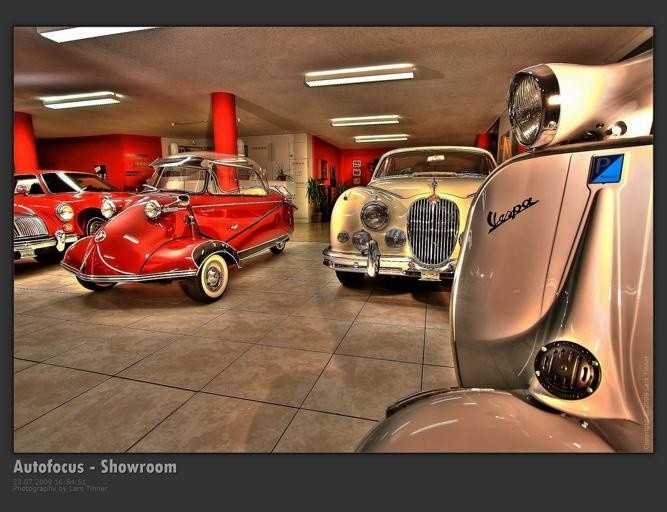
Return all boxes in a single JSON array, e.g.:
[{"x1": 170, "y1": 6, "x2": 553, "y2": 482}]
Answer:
[
  {"x1": 321, "y1": 145, "x2": 498, "y2": 289},
  {"x1": 14, "y1": 194, "x2": 84, "y2": 267},
  {"x1": 60, "y1": 151, "x2": 295, "y2": 303},
  {"x1": 14, "y1": 167, "x2": 144, "y2": 237},
  {"x1": 351, "y1": 49, "x2": 653, "y2": 454}
]
